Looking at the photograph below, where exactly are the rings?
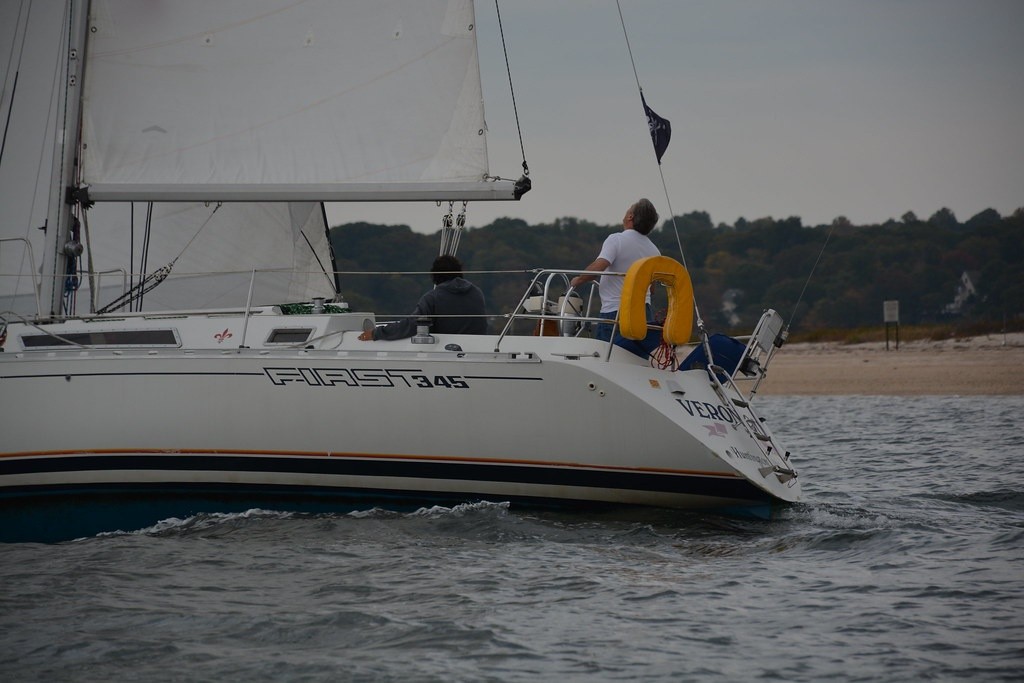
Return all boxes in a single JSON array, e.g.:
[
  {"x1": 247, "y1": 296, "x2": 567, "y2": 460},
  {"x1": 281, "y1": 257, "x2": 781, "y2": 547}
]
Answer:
[{"x1": 364, "y1": 336, "x2": 365, "y2": 338}]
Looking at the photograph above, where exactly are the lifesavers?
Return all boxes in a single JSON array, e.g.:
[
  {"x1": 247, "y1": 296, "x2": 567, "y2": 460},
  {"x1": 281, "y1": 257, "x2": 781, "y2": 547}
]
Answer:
[{"x1": 620, "y1": 255, "x2": 692, "y2": 343}]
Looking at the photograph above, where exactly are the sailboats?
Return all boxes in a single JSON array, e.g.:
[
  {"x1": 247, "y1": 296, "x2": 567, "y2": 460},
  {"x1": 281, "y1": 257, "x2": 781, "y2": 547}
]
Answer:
[{"x1": 0, "y1": 0, "x2": 807, "y2": 543}]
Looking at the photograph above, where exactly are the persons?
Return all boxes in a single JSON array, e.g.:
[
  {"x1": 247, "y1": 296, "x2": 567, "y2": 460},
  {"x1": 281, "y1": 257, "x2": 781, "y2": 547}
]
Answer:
[
  {"x1": 571, "y1": 198, "x2": 661, "y2": 341},
  {"x1": 357, "y1": 255, "x2": 488, "y2": 342}
]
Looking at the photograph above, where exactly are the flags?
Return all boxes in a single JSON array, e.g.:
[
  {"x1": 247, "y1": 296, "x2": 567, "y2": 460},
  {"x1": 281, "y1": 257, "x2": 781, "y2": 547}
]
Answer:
[{"x1": 641, "y1": 92, "x2": 671, "y2": 165}]
